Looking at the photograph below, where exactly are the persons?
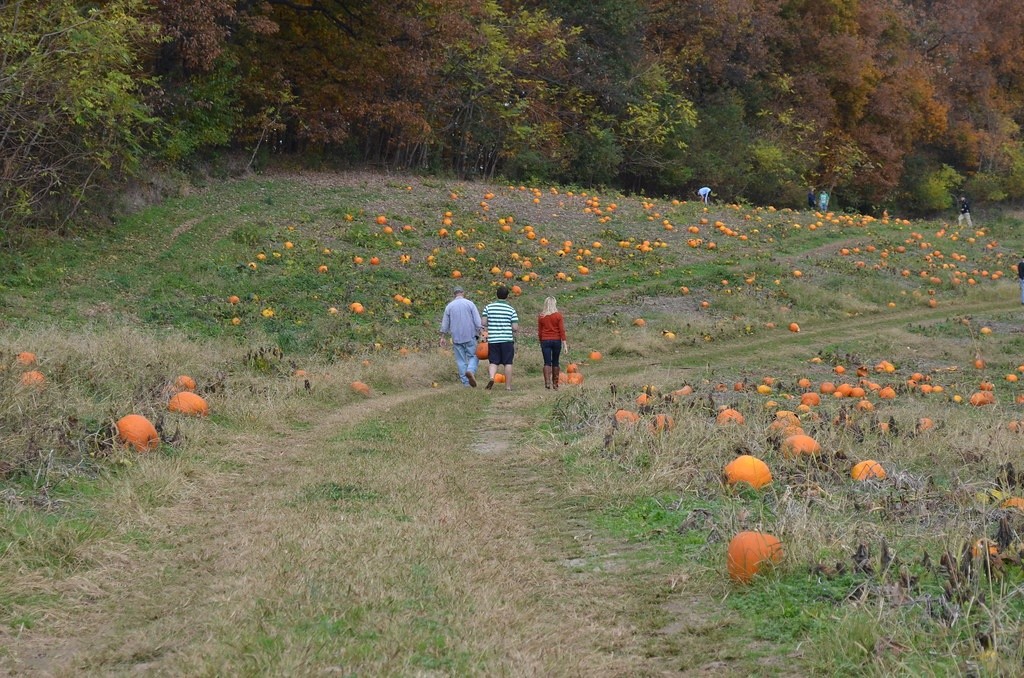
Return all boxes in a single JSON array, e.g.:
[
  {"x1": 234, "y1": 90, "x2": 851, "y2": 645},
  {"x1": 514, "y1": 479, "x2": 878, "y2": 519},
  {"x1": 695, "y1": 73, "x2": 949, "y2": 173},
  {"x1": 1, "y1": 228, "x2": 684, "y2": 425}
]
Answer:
[
  {"x1": 480, "y1": 285, "x2": 519, "y2": 391},
  {"x1": 537, "y1": 296, "x2": 568, "y2": 390},
  {"x1": 694, "y1": 187, "x2": 712, "y2": 203},
  {"x1": 957, "y1": 197, "x2": 973, "y2": 228},
  {"x1": 808, "y1": 188, "x2": 816, "y2": 210},
  {"x1": 1018, "y1": 256, "x2": 1024, "y2": 306},
  {"x1": 817, "y1": 189, "x2": 830, "y2": 213},
  {"x1": 439, "y1": 285, "x2": 484, "y2": 390}
]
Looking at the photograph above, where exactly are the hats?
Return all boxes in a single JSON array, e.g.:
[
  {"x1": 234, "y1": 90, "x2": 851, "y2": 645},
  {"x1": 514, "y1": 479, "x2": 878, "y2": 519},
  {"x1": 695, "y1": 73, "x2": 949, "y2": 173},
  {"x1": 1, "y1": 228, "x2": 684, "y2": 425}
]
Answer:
[{"x1": 711, "y1": 192, "x2": 717, "y2": 196}]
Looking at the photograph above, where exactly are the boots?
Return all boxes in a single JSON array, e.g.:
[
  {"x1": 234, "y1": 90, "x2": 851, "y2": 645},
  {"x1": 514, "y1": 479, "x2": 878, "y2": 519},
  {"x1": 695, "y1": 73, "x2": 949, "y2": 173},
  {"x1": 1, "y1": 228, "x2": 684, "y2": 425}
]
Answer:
[
  {"x1": 552, "y1": 367, "x2": 560, "y2": 389},
  {"x1": 543, "y1": 366, "x2": 553, "y2": 389}
]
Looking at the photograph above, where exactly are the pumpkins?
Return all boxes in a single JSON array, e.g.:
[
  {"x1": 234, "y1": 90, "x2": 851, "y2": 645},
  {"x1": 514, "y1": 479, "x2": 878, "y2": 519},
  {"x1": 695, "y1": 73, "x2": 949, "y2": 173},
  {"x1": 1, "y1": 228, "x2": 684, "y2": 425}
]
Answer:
[
  {"x1": 168, "y1": 391, "x2": 209, "y2": 417},
  {"x1": 228, "y1": 184, "x2": 1020, "y2": 396},
  {"x1": 612, "y1": 394, "x2": 1024, "y2": 586},
  {"x1": 115, "y1": 415, "x2": 158, "y2": 453},
  {"x1": 21, "y1": 370, "x2": 44, "y2": 387},
  {"x1": 20, "y1": 352, "x2": 35, "y2": 367},
  {"x1": 164, "y1": 377, "x2": 196, "y2": 394}
]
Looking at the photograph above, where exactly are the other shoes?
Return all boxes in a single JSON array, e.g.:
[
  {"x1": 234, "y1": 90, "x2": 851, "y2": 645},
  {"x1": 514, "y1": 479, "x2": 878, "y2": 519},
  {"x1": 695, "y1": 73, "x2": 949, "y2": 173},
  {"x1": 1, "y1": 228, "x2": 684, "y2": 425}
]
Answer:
[
  {"x1": 486, "y1": 379, "x2": 494, "y2": 389},
  {"x1": 505, "y1": 386, "x2": 512, "y2": 391},
  {"x1": 465, "y1": 371, "x2": 476, "y2": 387}
]
